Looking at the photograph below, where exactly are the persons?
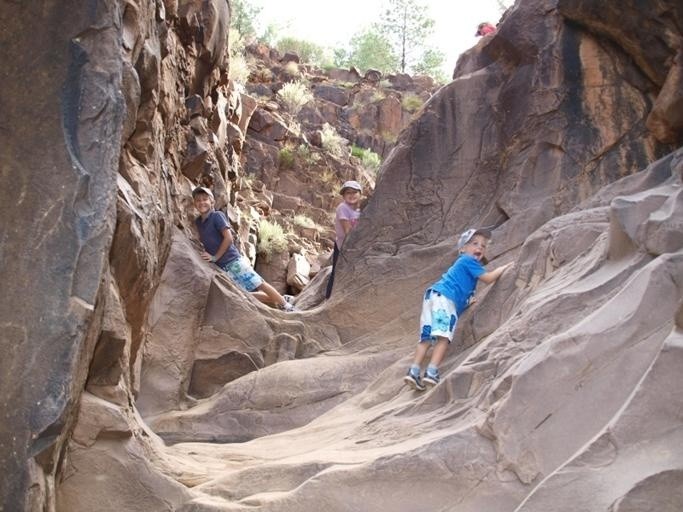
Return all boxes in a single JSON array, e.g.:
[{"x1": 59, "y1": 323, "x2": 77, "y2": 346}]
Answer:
[
  {"x1": 403, "y1": 229, "x2": 516, "y2": 391},
  {"x1": 325, "y1": 180, "x2": 363, "y2": 300},
  {"x1": 475, "y1": 22, "x2": 496, "y2": 36},
  {"x1": 190, "y1": 186, "x2": 299, "y2": 312}
]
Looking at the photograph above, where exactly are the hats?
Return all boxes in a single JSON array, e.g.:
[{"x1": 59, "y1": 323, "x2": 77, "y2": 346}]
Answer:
[
  {"x1": 457, "y1": 227, "x2": 492, "y2": 251},
  {"x1": 192, "y1": 186, "x2": 214, "y2": 199},
  {"x1": 338, "y1": 180, "x2": 362, "y2": 196}
]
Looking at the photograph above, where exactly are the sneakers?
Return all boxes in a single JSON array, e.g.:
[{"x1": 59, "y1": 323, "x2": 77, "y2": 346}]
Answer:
[
  {"x1": 279, "y1": 294, "x2": 301, "y2": 312},
  {"x1": 422, "y1": 369, "x2": 440, "y2": 385},
  {"x1": 404, "y1": 367, "x2": 426, "y2": 393}
]
